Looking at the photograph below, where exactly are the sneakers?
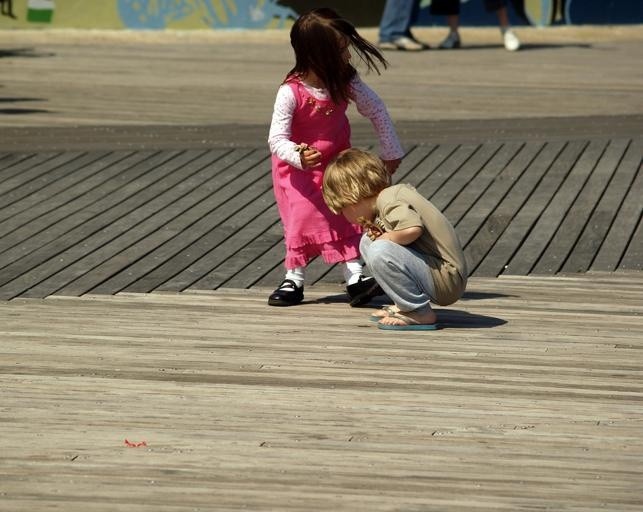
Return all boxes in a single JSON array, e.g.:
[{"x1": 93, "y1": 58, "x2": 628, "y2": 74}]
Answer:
[
  {"x1": 500, "y1": 29, "x2": 520, "y2": 52},
  {"x1": 375, "y1": 35, "x2": 429, "y2": 52},
  {"x1": 437, "y1": 33, "x2": 464, "y2": 49}
]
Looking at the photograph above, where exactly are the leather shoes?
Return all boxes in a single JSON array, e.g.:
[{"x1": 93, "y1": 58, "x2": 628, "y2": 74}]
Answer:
[
  {"x1": 345, "y1": 275, "x2": 385, "y2": 307},
  {"x1": 266, "y1": 278, "x2": 305, "y2": 307}
]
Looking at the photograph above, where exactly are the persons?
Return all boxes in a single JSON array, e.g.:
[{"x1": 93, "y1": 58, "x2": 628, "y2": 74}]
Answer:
[
  {"x1": 429, "y1": 1, "x2": 524, "y2": 50},
  {"x1": 376, "y1": 1, "x2": 431, "y2": 50},
  {"x1": 266, "y1": 6, "x2": 403, "y2": 307},
  {"x1": 320, "y1": 147, "x2": 468, "y2": 329}
]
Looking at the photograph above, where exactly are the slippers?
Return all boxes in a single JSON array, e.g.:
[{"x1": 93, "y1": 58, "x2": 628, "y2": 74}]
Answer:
[
  {"x1": 370, "y1": 305, "x2": 395, "y2": 323},
  {"x1": 377, "y1": 313, "x2": 439, "y2": 330}
]
